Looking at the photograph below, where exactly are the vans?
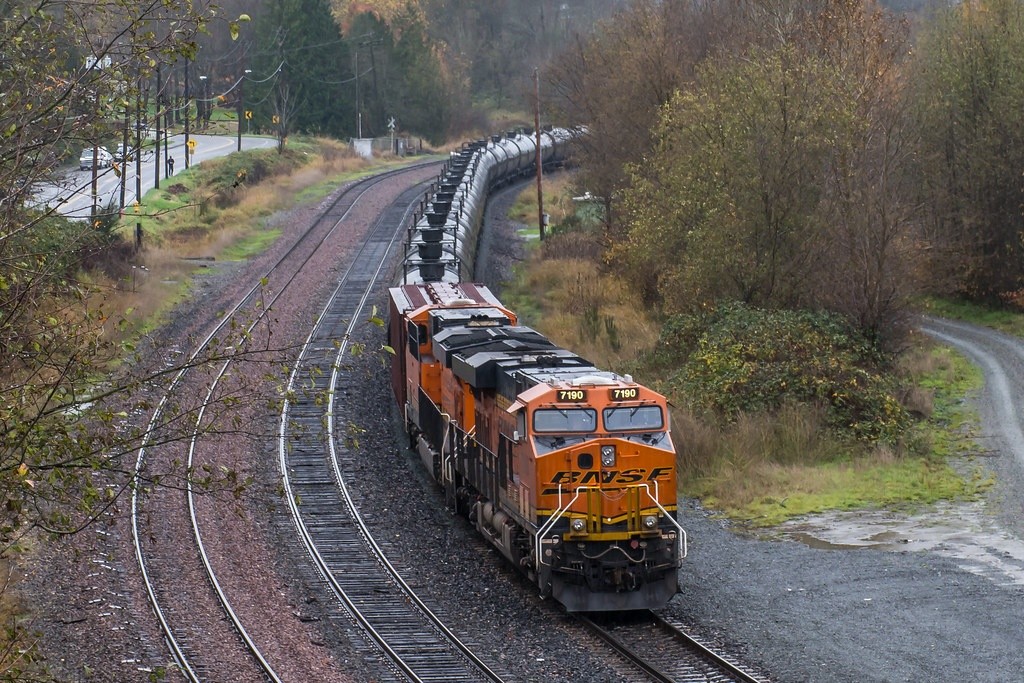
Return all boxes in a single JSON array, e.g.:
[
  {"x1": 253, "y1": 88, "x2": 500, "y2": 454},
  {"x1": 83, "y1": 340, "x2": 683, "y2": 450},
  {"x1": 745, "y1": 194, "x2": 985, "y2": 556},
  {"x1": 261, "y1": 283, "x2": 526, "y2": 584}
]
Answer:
[{"x1": 79, "y1": 147, "x2": 114, "y2": 171}]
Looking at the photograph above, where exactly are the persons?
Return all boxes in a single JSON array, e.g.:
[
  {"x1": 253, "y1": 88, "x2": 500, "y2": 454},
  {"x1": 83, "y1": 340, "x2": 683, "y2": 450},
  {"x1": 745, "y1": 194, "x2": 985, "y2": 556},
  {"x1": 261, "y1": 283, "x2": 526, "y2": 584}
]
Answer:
[{"x1": 168, "y1": 156, "x2": 174, "y2": 176}]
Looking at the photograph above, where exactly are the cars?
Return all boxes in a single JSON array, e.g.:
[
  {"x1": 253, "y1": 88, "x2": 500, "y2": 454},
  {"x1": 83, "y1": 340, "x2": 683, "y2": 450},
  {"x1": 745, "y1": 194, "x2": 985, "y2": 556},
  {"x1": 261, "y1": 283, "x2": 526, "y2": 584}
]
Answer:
[{"x1": 113, "y1": 146, "x2": 141, "y2": 162}]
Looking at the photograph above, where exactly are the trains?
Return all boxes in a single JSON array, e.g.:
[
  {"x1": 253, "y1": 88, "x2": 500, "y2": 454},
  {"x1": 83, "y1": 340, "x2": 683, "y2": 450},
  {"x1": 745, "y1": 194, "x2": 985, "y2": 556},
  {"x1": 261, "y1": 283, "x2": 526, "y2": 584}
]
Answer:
[{"x1": 387, "y1": 123, "x2": 688, "y2": 616}]
